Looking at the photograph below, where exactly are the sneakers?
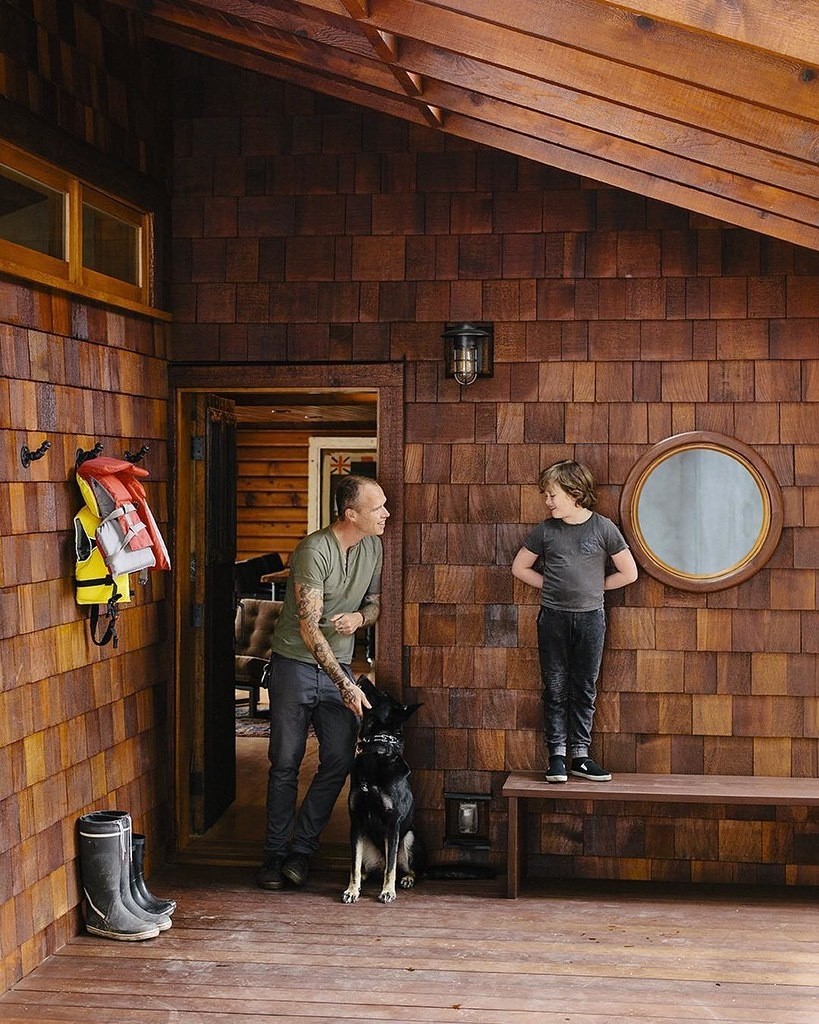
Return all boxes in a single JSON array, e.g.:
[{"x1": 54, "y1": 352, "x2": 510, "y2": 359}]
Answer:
[
  {"x1": 570, "y1": 758, "x2": 612, "y2": 781},
  {"x1": 545, "y1": 756, "x2": 568, "y2": 782}
]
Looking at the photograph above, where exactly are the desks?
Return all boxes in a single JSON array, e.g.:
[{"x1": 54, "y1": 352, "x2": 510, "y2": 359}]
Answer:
[{"x1": 260, "y1": 569, "x2": 291, "y2": 600}]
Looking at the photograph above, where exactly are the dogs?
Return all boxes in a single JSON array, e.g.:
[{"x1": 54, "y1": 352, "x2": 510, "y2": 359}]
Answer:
[{"x1": 341, "y1": 672, "x2": 420, "y2": 903}]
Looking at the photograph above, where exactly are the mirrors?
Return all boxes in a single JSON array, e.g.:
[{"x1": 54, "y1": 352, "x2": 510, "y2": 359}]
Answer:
[{"x1": 619, "y1": 430, "x2": 785, "y2": 594}]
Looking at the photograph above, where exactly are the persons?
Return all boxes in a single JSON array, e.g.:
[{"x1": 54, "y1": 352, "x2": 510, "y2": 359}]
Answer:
[
  {"x1": 513, "y1": 458, "x2": 637, "y2": 783},
  {"x1": 261, "y1": 474, "x2": 390, "y2": 890}
]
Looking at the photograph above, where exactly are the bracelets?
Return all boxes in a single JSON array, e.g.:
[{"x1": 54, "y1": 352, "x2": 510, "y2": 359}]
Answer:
[{"x1": 358, "y1": 610, "x2": 364, "y2": 627}]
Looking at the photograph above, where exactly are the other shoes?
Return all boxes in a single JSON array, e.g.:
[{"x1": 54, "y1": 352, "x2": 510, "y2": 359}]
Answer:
[
  {"x1": 283, "y1": 853, "x2": 311, "y2": 884},
  {"x1": 259, "y1": 865, "x2": 285, "y2": 890}
]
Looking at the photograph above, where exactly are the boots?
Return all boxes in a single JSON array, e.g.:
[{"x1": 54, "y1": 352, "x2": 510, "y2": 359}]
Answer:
[{"x1": 79, "y1": 811, "x2": 177, "y2": 941}]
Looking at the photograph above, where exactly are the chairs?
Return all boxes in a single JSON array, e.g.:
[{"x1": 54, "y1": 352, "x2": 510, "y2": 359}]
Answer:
[{"x1": 235, "y1": 598, "x2": 283, "y2": 717}]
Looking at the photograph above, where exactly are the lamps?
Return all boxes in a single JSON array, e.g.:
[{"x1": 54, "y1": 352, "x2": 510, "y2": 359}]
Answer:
[{"x1": 440, "y1": 322, "x2": 494, "y2": 386}]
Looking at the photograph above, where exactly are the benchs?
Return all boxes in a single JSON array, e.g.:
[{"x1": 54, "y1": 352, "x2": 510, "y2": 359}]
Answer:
[{"x1": 501, "y1": 769, "x2": 819, "y2": 899}]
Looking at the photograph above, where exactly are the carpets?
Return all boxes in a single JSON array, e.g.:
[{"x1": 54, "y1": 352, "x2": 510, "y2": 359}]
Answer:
[{"x1": 235, "y1": 706, "x2": 319, "y2": 738}]
